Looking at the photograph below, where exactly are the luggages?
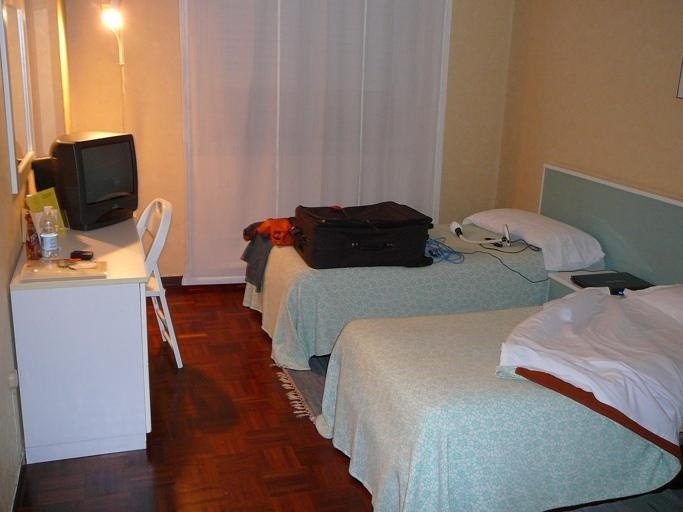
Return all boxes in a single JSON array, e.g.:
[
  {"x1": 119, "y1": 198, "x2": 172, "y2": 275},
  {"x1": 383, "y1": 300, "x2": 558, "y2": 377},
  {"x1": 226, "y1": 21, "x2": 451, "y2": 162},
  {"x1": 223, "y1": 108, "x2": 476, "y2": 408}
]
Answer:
[{"x1": 291, "y1": 201, "x2": 434, "y2": 270}]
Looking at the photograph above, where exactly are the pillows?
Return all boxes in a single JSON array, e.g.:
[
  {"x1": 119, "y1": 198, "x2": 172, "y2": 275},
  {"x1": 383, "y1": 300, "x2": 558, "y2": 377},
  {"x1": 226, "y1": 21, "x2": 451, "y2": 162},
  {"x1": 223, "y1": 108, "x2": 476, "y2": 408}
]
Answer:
[
  {"x1": 465, "y1": 206, "x2": 605, "y2": 273},
  {"x1": 624, "y1": 282, "x2": 681, "y2": 329}
]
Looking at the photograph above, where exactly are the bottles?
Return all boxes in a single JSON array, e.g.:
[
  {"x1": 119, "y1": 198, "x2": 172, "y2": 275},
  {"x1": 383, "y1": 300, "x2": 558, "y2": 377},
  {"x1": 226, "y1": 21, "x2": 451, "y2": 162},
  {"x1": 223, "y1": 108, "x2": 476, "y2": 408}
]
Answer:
[
  {"x1": 25, "y1": 213, "x2": 42, "y2": 260},
  {"x1": 40, "y1": 220, "x2": 60, "y2": 259}
]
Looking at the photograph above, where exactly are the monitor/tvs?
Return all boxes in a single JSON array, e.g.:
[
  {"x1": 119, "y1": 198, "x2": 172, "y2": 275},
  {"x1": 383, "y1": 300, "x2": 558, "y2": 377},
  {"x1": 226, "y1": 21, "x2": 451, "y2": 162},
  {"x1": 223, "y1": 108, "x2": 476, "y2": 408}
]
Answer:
[{"x1": 49, "y1": 131, "x2": 138, "y2": 231}]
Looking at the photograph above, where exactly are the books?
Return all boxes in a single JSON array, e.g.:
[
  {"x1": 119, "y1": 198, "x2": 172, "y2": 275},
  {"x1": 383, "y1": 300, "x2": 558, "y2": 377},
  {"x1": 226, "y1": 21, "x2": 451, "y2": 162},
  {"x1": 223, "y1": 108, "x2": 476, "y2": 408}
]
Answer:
[{"x1": 26, "y1": 187, "x2": 67, "y2": 246}]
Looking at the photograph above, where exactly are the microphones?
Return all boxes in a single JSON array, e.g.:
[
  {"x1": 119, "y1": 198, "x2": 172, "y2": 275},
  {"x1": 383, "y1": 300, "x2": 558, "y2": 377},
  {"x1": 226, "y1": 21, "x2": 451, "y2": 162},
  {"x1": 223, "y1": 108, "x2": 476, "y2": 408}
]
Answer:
[{"x1": 450, "y1": 221, "x2": 507, "y2": 244}]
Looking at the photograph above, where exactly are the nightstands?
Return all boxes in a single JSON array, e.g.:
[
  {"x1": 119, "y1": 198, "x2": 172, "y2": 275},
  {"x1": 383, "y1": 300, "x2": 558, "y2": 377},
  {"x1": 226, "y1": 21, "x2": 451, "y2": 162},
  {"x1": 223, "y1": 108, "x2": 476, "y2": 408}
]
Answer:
[{"x1": 544, "y1": 271, "x2": 652, "y2": 305}]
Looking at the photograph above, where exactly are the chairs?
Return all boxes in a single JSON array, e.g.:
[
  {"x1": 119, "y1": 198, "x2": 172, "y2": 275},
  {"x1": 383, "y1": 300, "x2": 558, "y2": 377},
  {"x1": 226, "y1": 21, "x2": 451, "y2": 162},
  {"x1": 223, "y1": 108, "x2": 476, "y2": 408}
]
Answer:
[{"x1": 136, "y1": 199, "x2": 185, "y2": 370}]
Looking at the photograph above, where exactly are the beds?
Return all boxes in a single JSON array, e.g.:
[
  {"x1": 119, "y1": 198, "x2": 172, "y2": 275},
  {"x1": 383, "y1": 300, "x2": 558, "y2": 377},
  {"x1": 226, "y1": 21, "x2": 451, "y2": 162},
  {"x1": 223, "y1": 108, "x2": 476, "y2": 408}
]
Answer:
[
  {"x1": 315, "y1": 280, "x2": 682, "y2": 509},
  {"x1": 240, "y1": 221, "x2": 607, "y2": 369}
]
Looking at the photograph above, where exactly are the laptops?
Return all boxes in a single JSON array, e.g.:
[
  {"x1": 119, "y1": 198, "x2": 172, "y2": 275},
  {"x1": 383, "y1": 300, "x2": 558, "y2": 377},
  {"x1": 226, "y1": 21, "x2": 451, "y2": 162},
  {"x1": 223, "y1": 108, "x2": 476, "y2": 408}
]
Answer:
[{"x1": 570, "y1": 272, "x2": 655, "y2": 294}]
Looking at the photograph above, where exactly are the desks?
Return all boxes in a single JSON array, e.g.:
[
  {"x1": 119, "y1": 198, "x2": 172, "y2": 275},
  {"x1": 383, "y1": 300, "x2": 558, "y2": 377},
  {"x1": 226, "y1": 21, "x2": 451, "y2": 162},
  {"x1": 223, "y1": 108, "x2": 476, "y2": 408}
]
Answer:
[{"x1": 7, "y1": 210, "x2": 154, "y2": 466}]
